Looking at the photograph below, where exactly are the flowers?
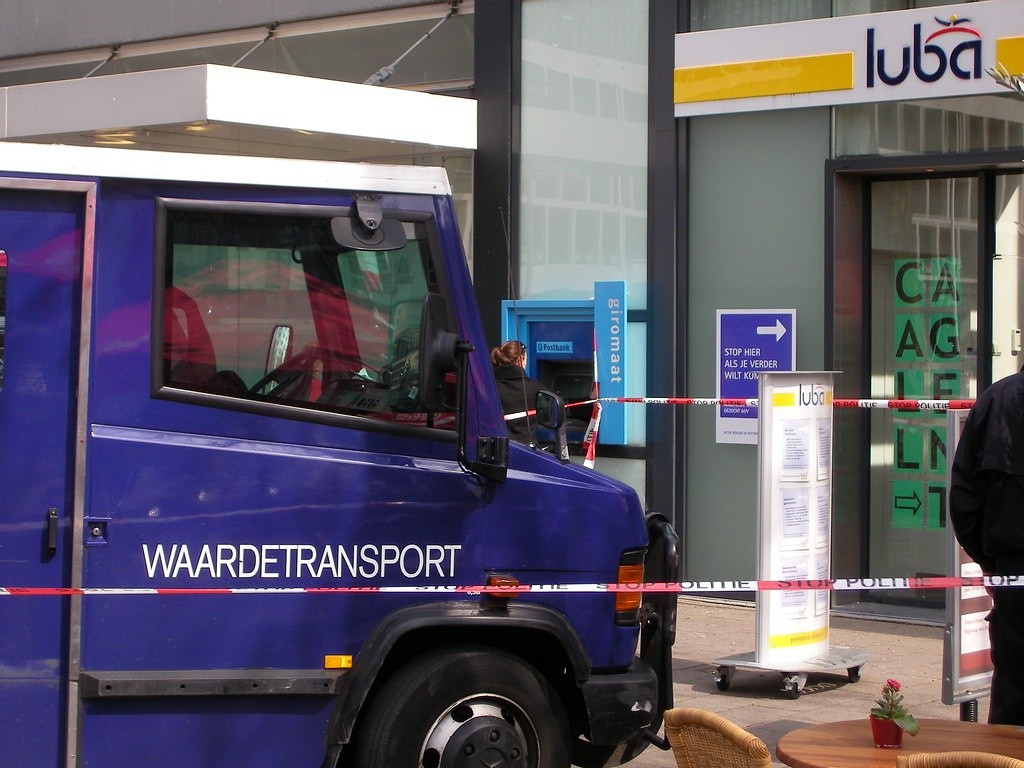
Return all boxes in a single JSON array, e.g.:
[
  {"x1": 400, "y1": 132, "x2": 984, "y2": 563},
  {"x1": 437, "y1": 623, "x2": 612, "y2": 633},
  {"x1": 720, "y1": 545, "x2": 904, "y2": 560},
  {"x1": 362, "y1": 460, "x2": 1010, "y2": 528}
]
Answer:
[{"x1": 870, "y1": 679, "x2": 920, "y2": 739}]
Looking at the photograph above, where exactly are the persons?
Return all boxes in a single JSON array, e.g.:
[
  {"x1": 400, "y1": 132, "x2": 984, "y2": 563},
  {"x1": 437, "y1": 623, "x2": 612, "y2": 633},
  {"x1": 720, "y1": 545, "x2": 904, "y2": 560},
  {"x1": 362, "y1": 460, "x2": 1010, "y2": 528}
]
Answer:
[
  {"x1": 491, "y1": 341, "x2": 550, "y2": 447},
  {"x1": 949, "y1": 364, "x2": 1024, "y2": 727}
]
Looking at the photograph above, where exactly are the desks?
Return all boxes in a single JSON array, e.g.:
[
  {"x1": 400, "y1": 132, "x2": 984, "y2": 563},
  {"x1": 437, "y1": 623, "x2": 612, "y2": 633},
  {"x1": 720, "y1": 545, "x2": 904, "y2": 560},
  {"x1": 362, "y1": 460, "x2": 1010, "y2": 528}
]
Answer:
[{"x1": 775, "y1": 717, "x2": 1024, "y2": 768}]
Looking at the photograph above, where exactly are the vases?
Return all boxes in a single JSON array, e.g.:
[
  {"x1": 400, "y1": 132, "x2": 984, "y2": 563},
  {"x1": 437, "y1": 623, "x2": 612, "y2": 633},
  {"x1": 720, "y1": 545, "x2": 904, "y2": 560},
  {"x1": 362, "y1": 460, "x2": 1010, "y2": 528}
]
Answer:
[{"x1": 869, "y1": 714, "x2": 904, "y2": 749}]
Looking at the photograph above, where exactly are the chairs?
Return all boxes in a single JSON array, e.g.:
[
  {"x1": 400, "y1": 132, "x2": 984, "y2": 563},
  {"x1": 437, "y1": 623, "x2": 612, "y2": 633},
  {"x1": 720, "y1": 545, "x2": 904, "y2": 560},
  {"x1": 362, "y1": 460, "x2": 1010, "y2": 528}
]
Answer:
[{"x1": 663, "y1": 707, "x2": 774, "y2": 768}]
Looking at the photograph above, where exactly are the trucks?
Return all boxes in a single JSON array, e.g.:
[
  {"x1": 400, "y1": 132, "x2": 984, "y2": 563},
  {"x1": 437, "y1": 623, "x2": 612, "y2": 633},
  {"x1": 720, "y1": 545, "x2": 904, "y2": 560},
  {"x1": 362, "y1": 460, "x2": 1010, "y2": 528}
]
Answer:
[{"x1": 0, "y1": 137, "x2": 681, "y2": 768}]
